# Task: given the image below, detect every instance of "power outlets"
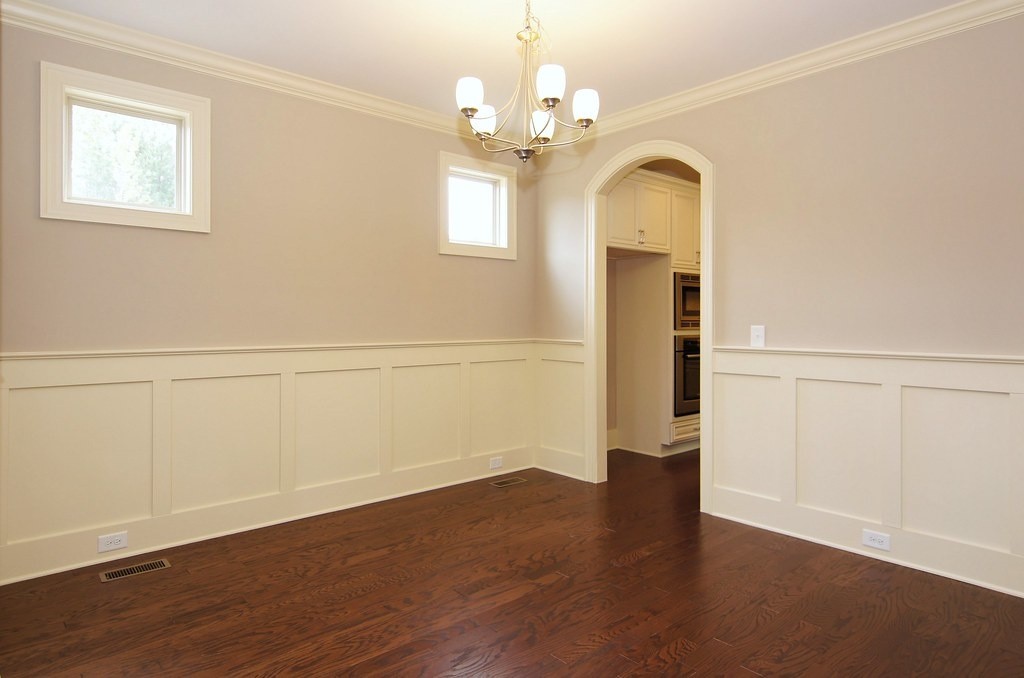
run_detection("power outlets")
[863,529,890,551]
[98,531,128,553]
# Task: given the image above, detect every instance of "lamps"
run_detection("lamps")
[456,0,600,162]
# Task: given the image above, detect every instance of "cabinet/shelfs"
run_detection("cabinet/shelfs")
[607,168,671,259]
[661,414,700,458]
[671,177,700,274]
